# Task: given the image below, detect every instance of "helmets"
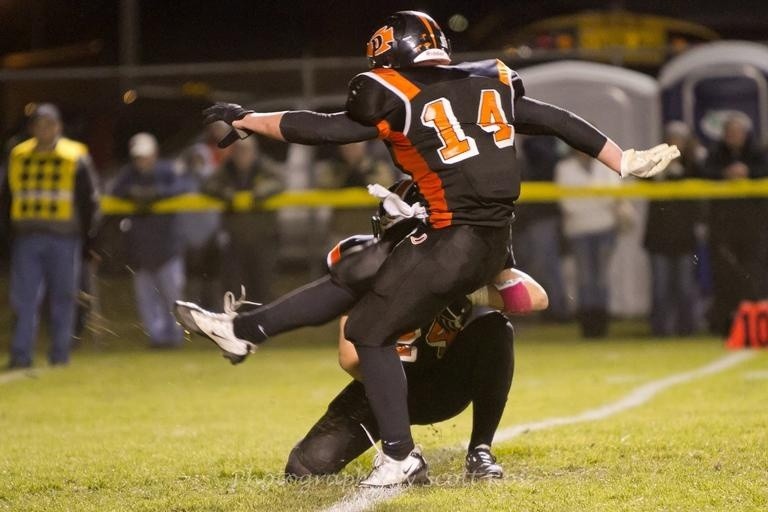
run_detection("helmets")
[364,10,452,67]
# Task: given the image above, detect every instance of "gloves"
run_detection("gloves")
[619,142,681,180]
[200,101,256,150]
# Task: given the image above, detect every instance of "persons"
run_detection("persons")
[555,138,621,340]
[2,101,98,372]
[170,9,681,484]
[703,111,767,337]
[283,175,550,481]
[99,131,199,352]
[220,134,287,304]
[635,119,707,338]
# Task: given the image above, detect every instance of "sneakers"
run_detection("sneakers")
[464,444,505,481]
[170,300,265,367]
[362,442,431,489]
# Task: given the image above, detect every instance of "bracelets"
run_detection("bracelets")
[465,286,490,307]
